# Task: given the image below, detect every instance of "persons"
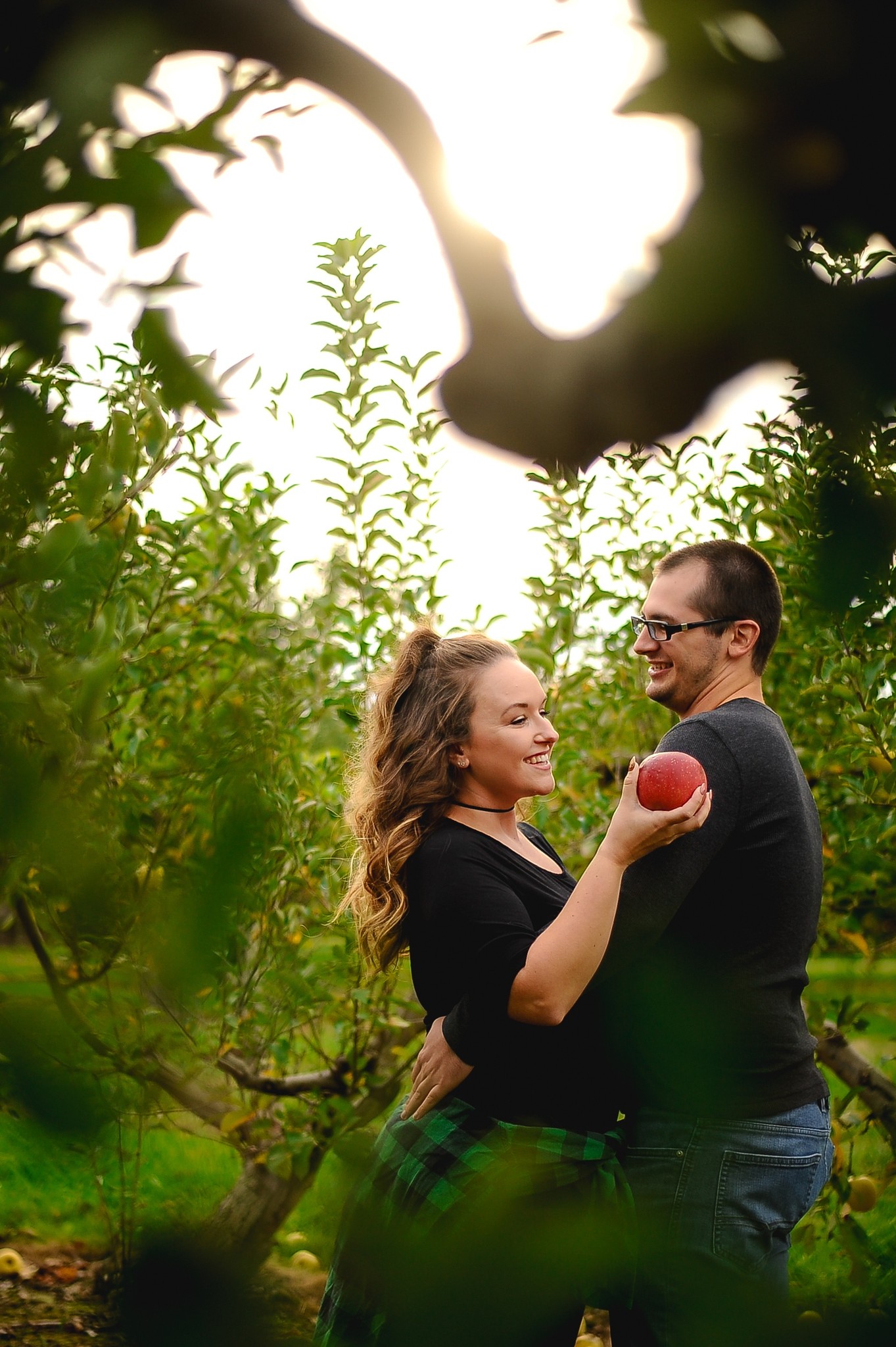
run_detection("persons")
[401,539,836,1347]
[310,629,714,1346]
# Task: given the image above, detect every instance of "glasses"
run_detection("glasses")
[631,616,747,642]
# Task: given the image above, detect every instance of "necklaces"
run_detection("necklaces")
[451,799,517,813]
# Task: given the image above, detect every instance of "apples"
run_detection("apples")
[573,1316,605,1347]
[828,1120,844,1175]
[796,1311,824,1328]
[0,1248,23,1279]
[62,504,138,533]
[846,1176,877,1212]
[135,862,166,891]
[285,1232,318,1273]
[636,752,707,816]
